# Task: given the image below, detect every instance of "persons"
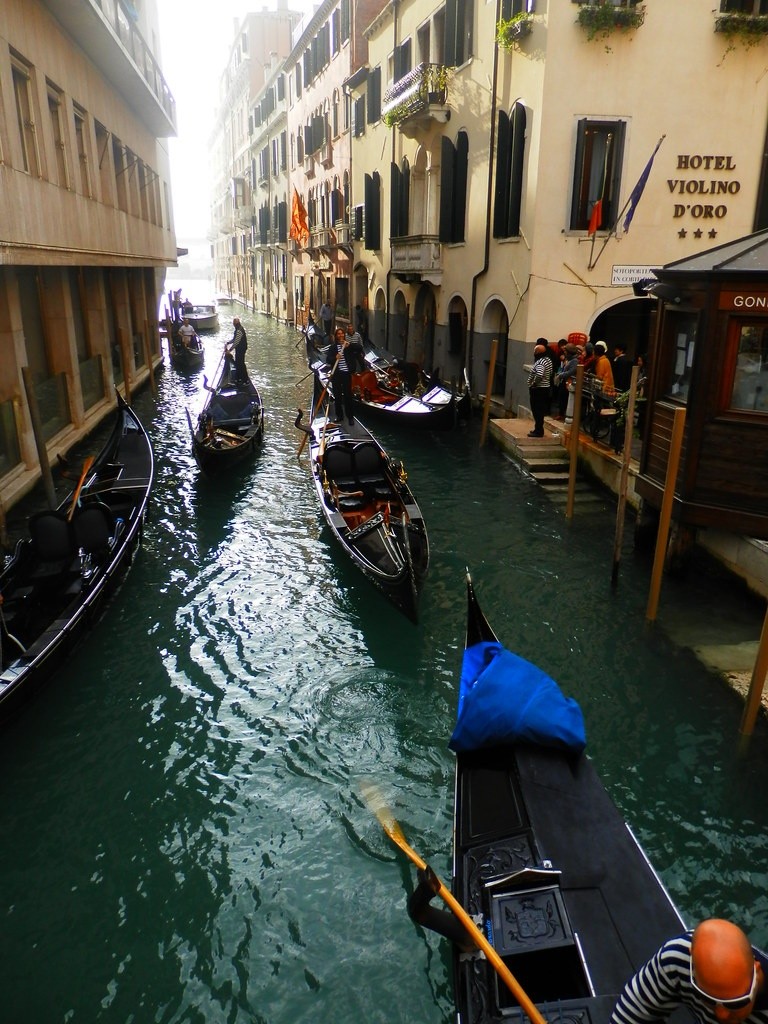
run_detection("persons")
[320,299,333,336]
[356,305,367,337]
[535,338,648,449]
[183,298,193,313]
[226,318,250,386]
[178,319,194,347]
[609,918,768,1024]
[527,345,553,437]
[345,325,363,373]
[326,327,363,426]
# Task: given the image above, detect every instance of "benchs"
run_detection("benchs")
[323,442,392,510]
[28,502,116,579]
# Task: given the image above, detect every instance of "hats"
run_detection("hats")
[576,345,583,354]
[565,343,577,352]
[595,341,608,353]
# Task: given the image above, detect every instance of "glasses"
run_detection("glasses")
[687,953,759,1010]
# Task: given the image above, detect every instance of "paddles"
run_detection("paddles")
[297,341,346,460]
[295,362,327,386]
[390,831,547,1024]
[194,347,227,430]
[295,317,322,348]
[317,404,329,463]
[69,456,95,521]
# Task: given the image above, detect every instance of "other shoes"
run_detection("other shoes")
[553,415,565,420]
[334,417,344,423]
[348,417,355,426]
[241,381,250,387]
[527,430,544,438]
[234,379,242,383]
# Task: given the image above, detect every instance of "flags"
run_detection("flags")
[623,147,657,235]
[588,154,607,236]
[289,189,309,248]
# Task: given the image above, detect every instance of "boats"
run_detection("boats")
[1,386,155,710]
[293,361,433,624]
[191,343,267,466]
[182,301,219,332]
[406,566,700,1024]
[170,330,207,368]
[297,305,476,427]
